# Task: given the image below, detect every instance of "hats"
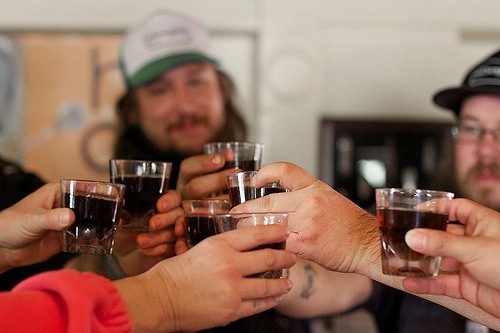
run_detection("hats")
[118,13,237,95]
[431,48,500,117]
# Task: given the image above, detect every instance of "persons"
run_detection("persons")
[0,224,298,333]
[0,153,125,291]
[111,13,500,333]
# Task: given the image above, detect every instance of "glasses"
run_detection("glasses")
[450,124,500,146]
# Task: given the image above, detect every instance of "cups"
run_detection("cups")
[181,198,231,247]
[373,187,454,279]
[58,178,126,256]
[212,210,290,284]
[227,170,288,207]
[108,157,173,233]
[203,142,263,199]
